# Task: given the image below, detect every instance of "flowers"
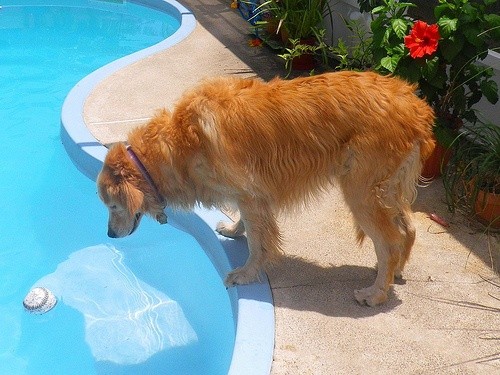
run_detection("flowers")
[230,0,263,48]
[373,0,500,137]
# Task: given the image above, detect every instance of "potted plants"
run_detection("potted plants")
[440,120,500,229]
[265,0,323,71]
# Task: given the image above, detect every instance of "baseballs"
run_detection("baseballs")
[23,286,57,315]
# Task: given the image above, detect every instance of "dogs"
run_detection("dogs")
[97,71,437,307]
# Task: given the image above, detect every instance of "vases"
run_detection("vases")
[419,139,455,180]
